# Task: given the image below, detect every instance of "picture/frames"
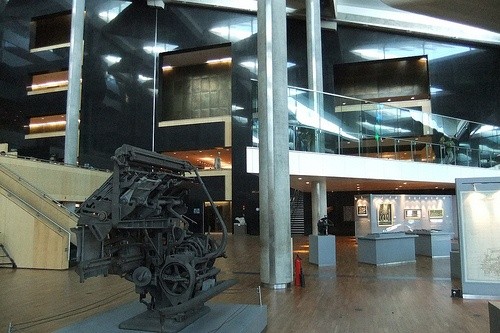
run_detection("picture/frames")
[404,209,423,220]
[459,190,500,284]
[375,202,394,228]
[357,204,368,217]
[428,209,445,219]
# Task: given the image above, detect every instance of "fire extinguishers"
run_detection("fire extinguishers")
[295,256,302,287]
